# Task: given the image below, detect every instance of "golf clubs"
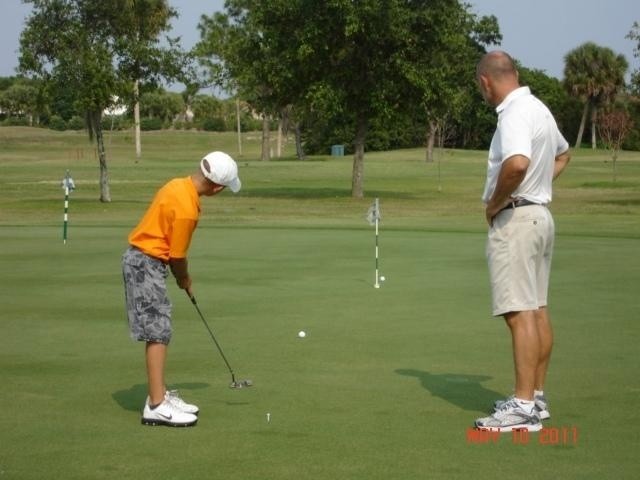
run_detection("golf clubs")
[186,285,253,390]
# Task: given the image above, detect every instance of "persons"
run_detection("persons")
[473,51,572,433]
[121,152,242,427]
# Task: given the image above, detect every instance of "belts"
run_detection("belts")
[503,199,534,209]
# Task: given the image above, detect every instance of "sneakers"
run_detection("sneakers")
[475,400,543,432]
[142,399,198,427]
[145,389,200,414]
[493,394,551,420]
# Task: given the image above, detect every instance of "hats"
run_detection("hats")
[200,151,241,193]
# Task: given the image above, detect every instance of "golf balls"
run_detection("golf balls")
[299,331,306,337]
[380,275,384,281]
